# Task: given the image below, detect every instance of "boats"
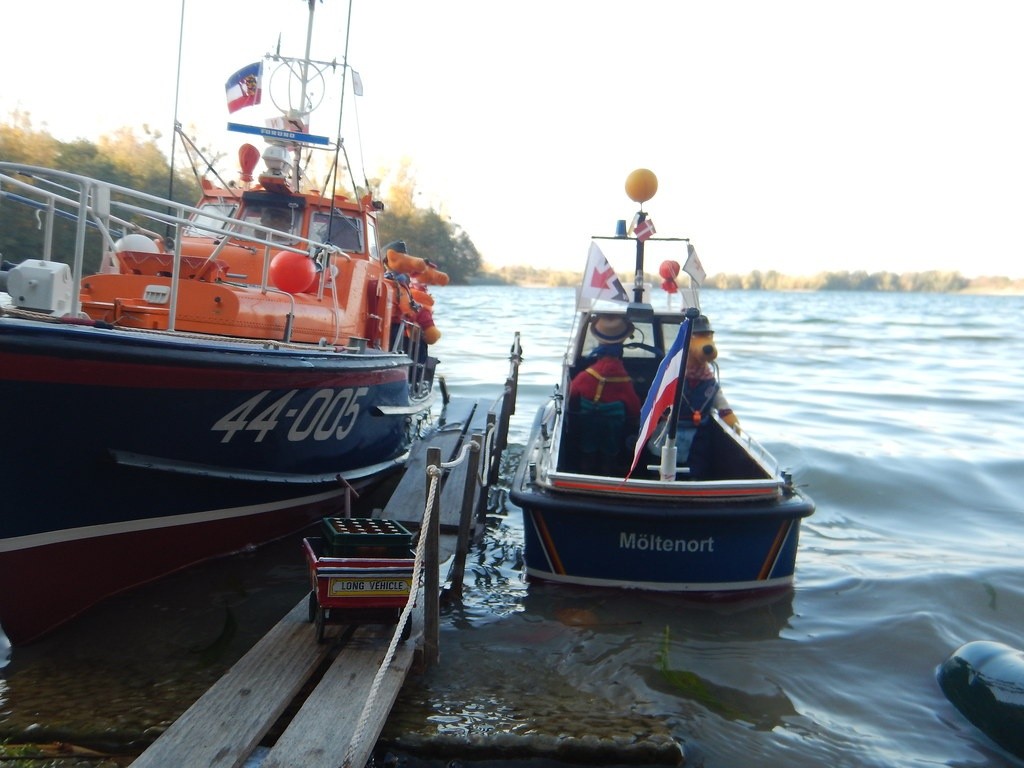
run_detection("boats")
[0,0,444,647]
[508,167,818,601]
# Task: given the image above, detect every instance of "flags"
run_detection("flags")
[684,245,707,286]
[225,62,263,114]
[581,242,633,309]
[622,320,690,485]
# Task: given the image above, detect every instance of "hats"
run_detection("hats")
[380,241,407,262]
[591,314,635,344]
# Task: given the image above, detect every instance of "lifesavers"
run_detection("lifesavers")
[275,115,307,152]
